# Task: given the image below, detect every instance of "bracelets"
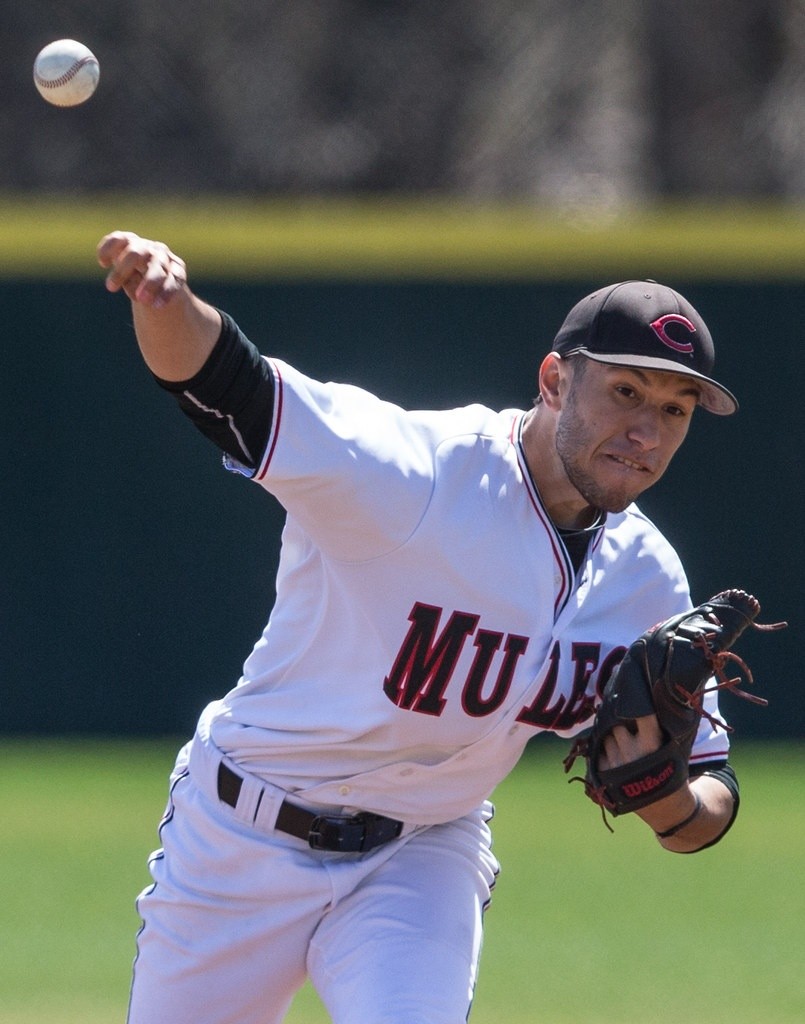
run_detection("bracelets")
[654,784,706,845]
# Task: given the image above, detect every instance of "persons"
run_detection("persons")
[95,227,740,1024]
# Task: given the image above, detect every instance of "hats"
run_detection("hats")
[552,279,739,417]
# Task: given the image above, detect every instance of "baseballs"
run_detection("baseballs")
[33,38,101,107]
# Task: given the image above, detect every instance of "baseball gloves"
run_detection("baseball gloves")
[562,589,788,834]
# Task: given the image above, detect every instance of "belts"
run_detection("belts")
[216,762,404,853]
